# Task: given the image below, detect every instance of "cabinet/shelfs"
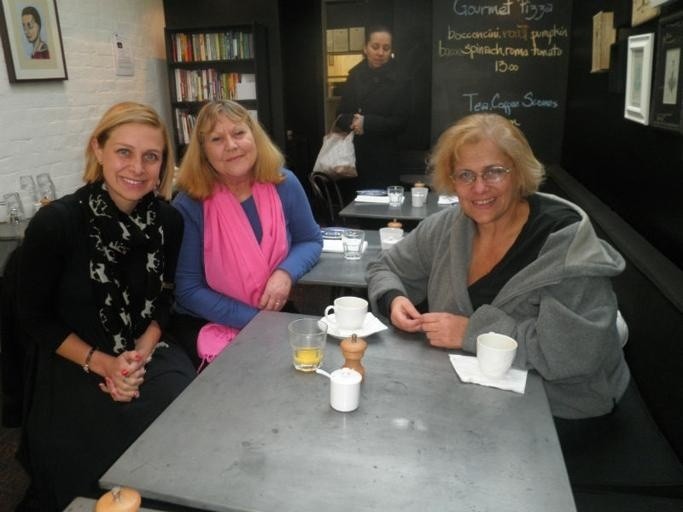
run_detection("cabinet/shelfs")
[162,20,278,171]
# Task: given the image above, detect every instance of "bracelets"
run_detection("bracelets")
[82,345,96,375]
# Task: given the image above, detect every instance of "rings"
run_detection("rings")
[273,301,282,307]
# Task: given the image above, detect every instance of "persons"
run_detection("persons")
[364,113,631,484]
[166,100,323,367]
[3,101,197,497]
[19,5,51,60]
[335,20,416,188]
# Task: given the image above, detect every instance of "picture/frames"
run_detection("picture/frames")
[623,31,654,127]
[652,11,682,131]
[1,0,71,87]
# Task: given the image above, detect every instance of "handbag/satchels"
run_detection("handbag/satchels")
[311,112,360,180]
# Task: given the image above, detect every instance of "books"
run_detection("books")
[173,29,257,146]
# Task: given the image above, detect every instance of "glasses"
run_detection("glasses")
[447,161,517,184]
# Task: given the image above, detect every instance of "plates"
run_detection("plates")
[318,315,374,340]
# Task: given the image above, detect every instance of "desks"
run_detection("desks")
[336,191,459,234]
[295,225,414,310]
[96,308,579,512]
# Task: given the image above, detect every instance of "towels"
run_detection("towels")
[316,234,376,260]
[354,193,404,206]
[434,192,460,207]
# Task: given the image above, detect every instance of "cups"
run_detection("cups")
[330,367,363,412]
[288,317,329,371]
[475,332,519,379]
[323,295,368,331]
[340,230,365,260]
[387,185,403,206]
[413,188,430,207]
[379,227,404,252]
